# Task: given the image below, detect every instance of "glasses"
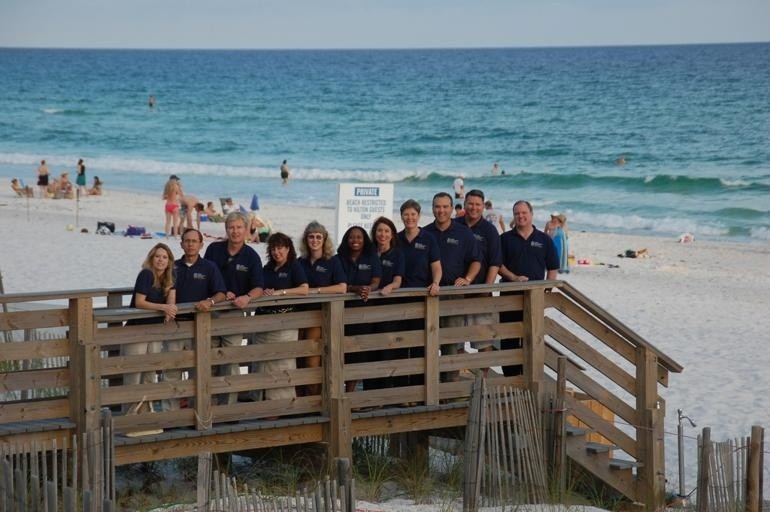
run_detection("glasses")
[308,235,324,240]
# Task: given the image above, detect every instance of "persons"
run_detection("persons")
[11,158,103,199]
[618,247,648,258]
[149,93,154,111]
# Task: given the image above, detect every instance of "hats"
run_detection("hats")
[169,174,180,180]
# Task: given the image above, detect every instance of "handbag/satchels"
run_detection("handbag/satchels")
[121,395,164,438]
